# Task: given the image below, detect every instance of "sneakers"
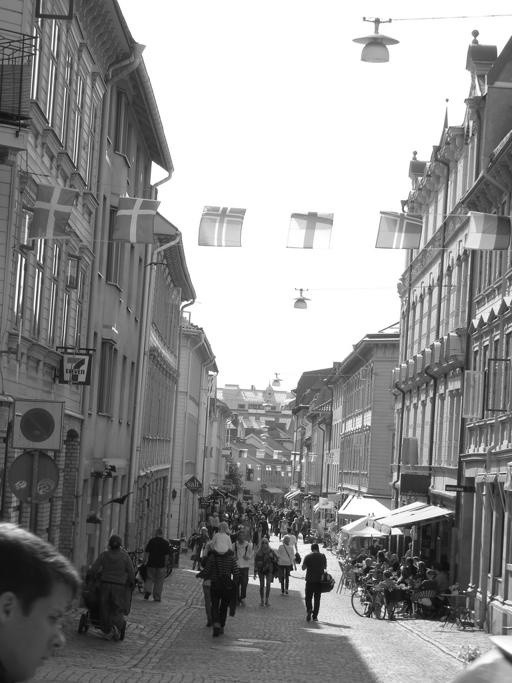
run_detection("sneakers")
[207,622,212,627]
[214,626,220,637]
[306,609,318,621]
[144,592,151,599]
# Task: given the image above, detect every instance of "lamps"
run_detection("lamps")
[294,284,456,309]
[352,13,511,63]
[272,372,355,387]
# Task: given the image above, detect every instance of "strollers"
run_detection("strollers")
[76,565,128,644]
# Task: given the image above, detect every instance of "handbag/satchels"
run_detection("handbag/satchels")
[320,573,335,592]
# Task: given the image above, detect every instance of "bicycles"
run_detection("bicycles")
[164,543,181,579]
[349,575,389,620]
[329,539,346,560]
[127,547,151,593]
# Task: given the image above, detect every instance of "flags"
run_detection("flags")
[112,193,160,244]
[466,212,509,249]
[375,209,428,252]
[29,184,77,241]
[286,207,337,253]
[196,203,249,247]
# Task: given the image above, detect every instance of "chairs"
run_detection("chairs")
[336,553,474,631]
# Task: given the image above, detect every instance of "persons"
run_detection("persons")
[188,499,451,634]
[88,535,136,640]
[140,528,171,603]
[0,523,83,683]
